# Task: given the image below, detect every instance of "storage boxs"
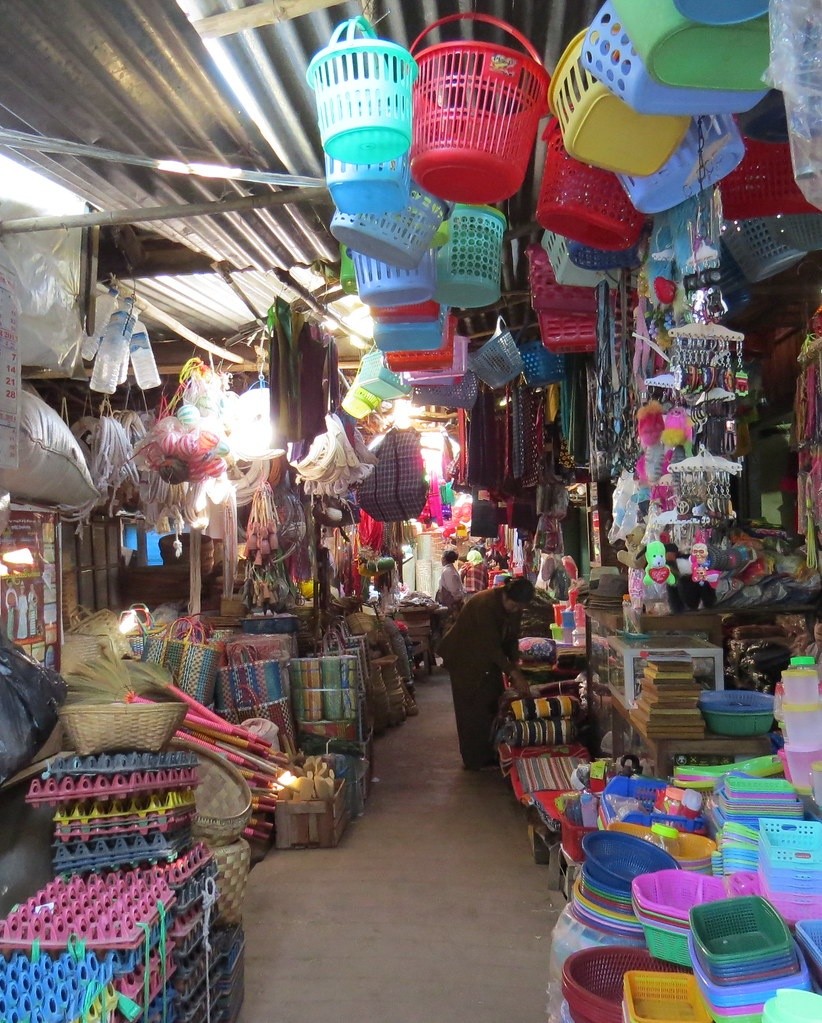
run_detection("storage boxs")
[266,778,351,848]
[607,633,724,712]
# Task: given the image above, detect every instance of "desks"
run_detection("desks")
[583,607,723,721]
[609,695,771,779]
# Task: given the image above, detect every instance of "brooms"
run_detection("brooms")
[62,647,293,842]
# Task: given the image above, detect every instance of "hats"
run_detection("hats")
[580,563,628,611]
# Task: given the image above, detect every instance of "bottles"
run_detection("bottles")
[642,823,680,857]
[580,795,598,827]
[81,289,161,394]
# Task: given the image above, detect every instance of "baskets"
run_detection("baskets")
[552,691,822,1023]
[62,632,104,673]
[58,699,188,757]
[163,735,251,850]
[303,0,822,420]
[209,839,251,927]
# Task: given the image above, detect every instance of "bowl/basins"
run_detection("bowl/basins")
[778,669,822,805]
[701,710,774,736]
[241,617,294,633]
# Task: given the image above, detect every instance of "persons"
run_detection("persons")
[464,549,489,603]
[437,574,536,774]
[469,539,492,569]
[438,550,465,668]
[317,541,338,582]
[496,549,511,570]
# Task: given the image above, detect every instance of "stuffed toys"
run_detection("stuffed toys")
[643,540,677,586]
[633,401,696,511]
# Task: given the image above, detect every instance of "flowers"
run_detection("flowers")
[519,638,552,658]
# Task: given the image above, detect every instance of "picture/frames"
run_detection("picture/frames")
[0,572,45,645]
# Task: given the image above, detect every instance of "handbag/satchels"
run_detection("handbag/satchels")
[354,425,430,525]
[312,497,359,529]
[440,587,465,607]
[69,603,373,743]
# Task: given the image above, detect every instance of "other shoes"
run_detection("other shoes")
[461,755,501,771]
